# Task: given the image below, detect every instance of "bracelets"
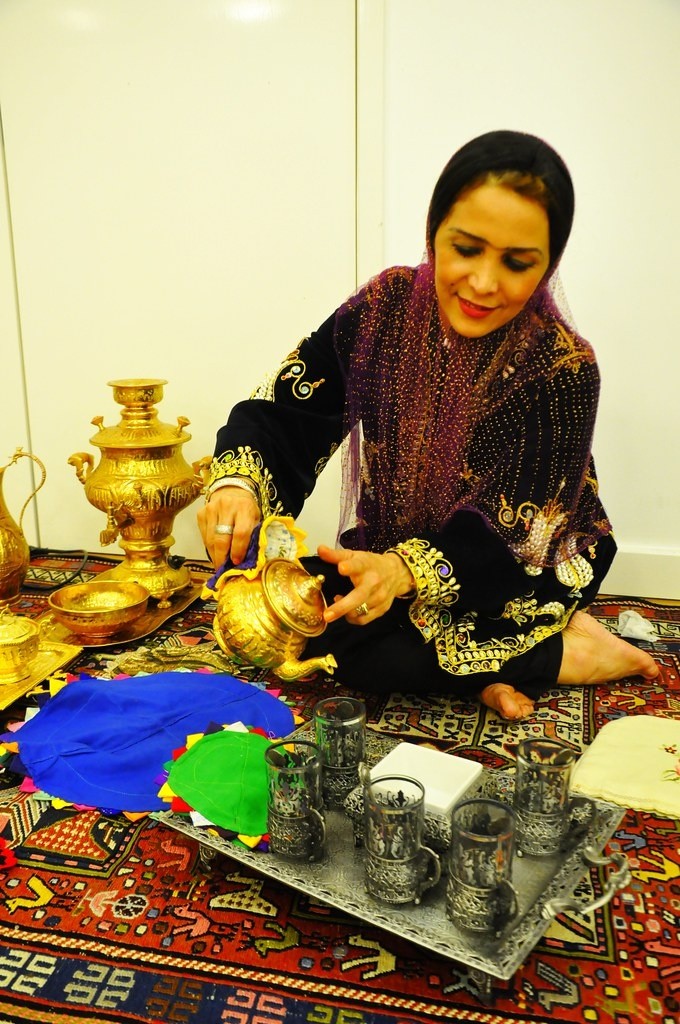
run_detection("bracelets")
[205,477,259,504]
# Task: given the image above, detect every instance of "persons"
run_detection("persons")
[196,132,660,722]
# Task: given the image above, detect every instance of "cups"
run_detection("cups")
[446,800,518,933]
[314,697,367,812]
[515,739,596,855]
[364,775,440,906]
[265,740,327,862]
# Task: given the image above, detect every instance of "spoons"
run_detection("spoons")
[269,749,288,802]
[554,748,574,777]
[334,701,354,721]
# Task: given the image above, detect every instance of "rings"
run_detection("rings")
[214,525,233,536]
[355,603,368,617]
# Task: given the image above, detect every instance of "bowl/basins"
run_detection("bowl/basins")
[49,585,149,637]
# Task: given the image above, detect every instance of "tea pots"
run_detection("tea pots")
[213,559,337,681]
